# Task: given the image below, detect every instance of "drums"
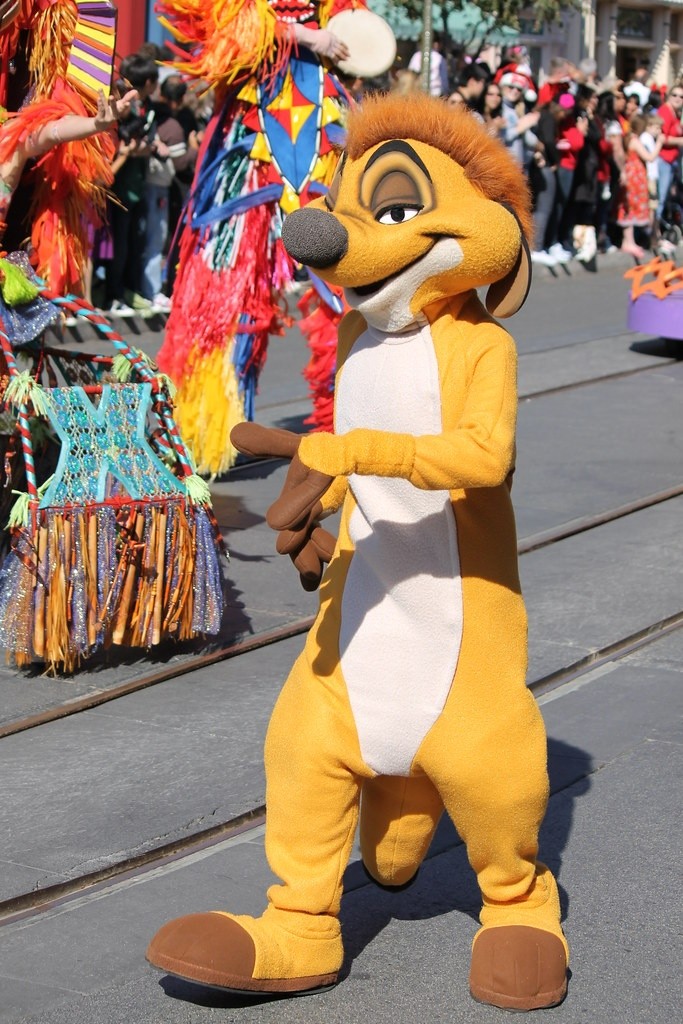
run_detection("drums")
[326,8,398,79]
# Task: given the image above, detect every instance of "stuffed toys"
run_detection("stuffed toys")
[147,97,571,1010]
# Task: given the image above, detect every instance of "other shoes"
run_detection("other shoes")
[531,242,572,267]
[621,244,645,257]
[110,290,172,318]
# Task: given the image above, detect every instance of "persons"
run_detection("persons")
[78,30,683,316]
[154,0,366,375]
[0,0,141,302]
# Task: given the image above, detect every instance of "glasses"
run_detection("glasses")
[508,85,522,91]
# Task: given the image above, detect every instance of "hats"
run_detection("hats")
[498,64,537,102]
